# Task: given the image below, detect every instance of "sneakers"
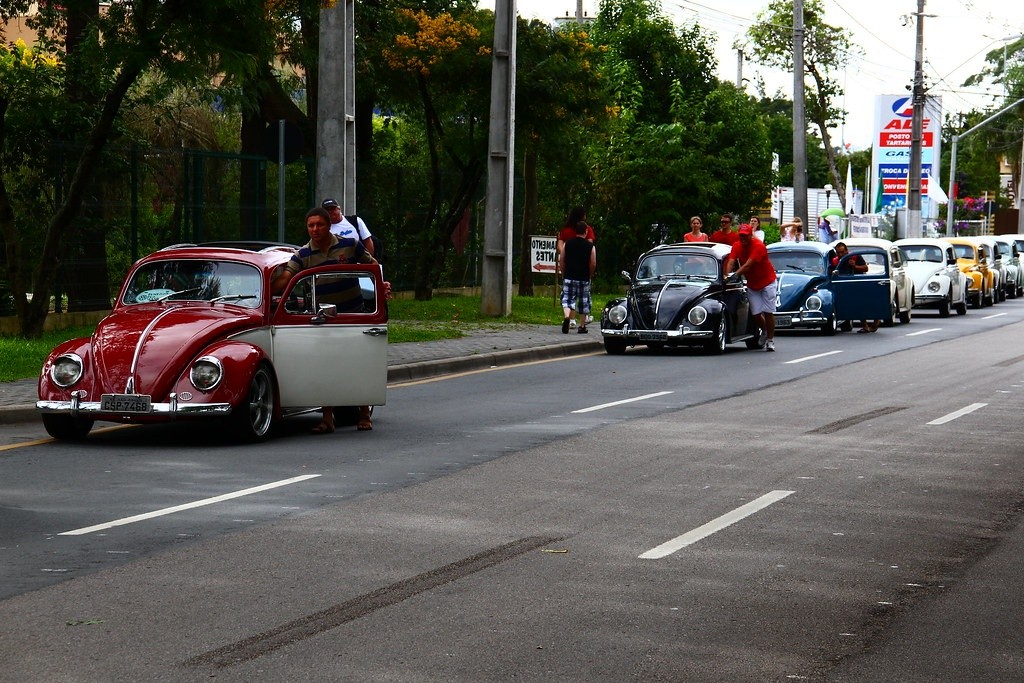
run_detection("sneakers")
[758,331,768,345]
[767,341,775,351]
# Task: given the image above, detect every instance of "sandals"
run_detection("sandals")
[357,415,372,430]
[310,421,335,434]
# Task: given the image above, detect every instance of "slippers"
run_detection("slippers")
[857,328,870,333]
[870,321,881,332]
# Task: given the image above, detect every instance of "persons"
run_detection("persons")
[684,217,708,263]
[819,218,838,244]
[558,206,594,329]
[560,221,596,334]
[710,215,738,248]
[829,243,880,332]
[747,217,766,246]
[779,217,804,243]
[268,199,391,432]
[724,224,777,351]
[320,199,374,262]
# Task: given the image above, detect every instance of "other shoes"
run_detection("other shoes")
[562,317,570,333]
[569,322,575,328]
[586,316,593,325]
[578,326,588,333]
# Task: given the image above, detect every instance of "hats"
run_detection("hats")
[738,224,752,235]
[322,199,339,208]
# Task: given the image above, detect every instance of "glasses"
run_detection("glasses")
[721,220,731,224]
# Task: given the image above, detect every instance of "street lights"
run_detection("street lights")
[824,185,833,209]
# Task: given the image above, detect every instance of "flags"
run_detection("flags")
[845,164,853,214]
[927,174,948,204]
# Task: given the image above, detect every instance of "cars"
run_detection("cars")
[600,241,765,356]
[35,239,387,443]
[763,240,891,336]
[829,234,1024,324]
